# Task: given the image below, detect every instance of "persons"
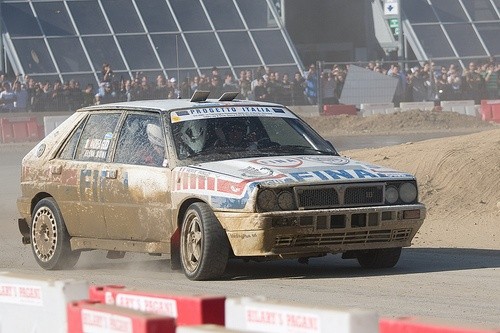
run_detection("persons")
[127,128,169,168]
[214,123,268,157]
[2,57,500,108]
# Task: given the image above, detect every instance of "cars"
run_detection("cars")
[16,89,427,281]
